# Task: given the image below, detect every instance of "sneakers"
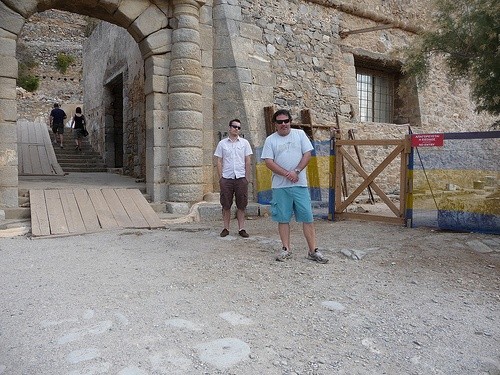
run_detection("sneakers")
[307,248,329,264]
[276,246,293,261]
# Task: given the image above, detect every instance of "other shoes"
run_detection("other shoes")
[220,229,229,237]
[79,148,82,151]
[239,229,249,237]
[53,140,58,144]
[73,145,79,151]
[60,144,64,148]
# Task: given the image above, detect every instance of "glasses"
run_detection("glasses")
[275,118,290,124]
[229,125,241,130]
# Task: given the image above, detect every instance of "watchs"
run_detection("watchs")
[294,168,300,173]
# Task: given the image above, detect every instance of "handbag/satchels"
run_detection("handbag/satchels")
[80,123,89,137]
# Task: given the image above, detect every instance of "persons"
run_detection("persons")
[49,103,67,148]
[261,109,329,264]
[214,119,253,238]
[70,106,86,151]
